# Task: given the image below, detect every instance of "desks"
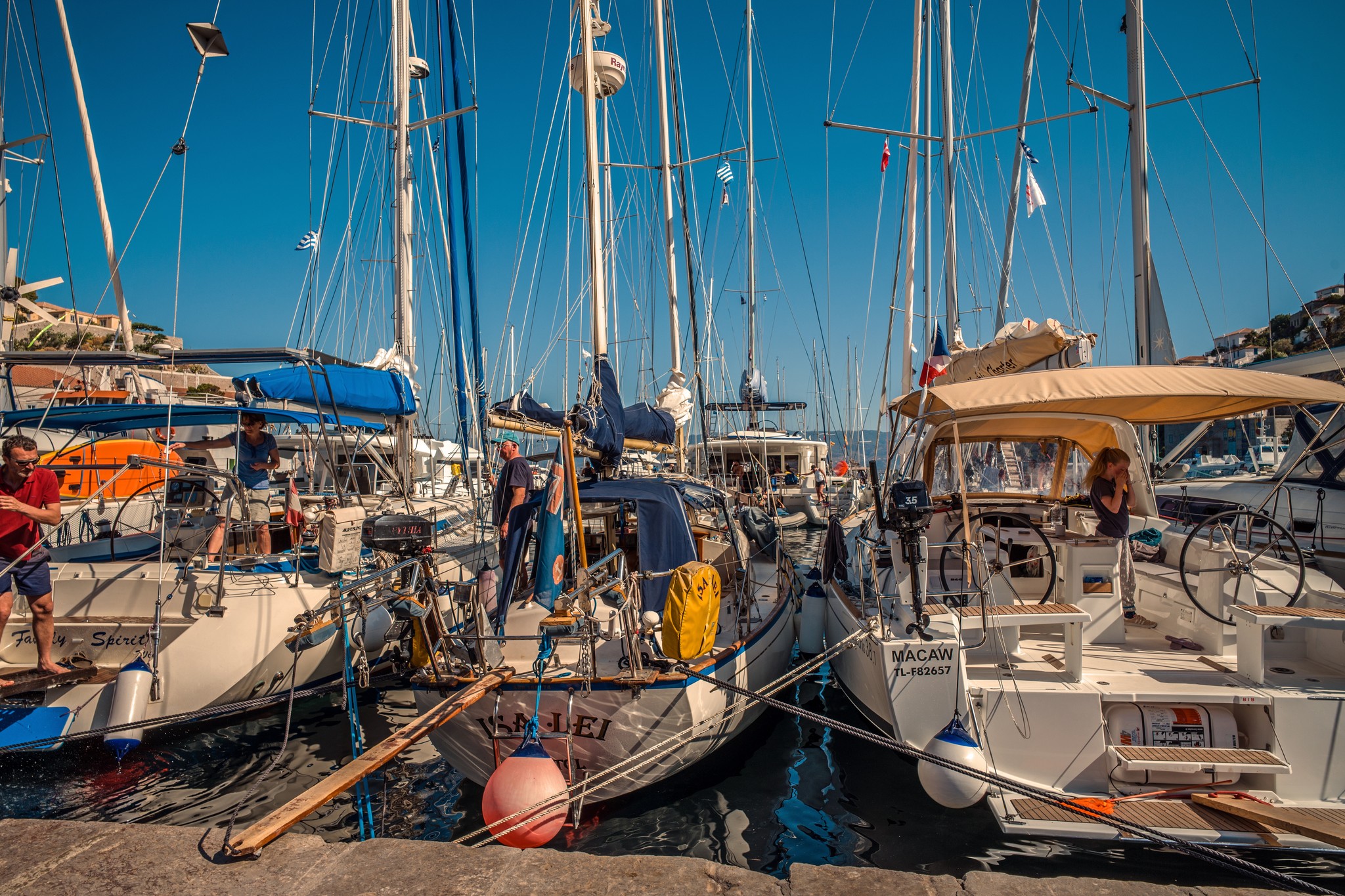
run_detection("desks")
[1039,526,1127,647]
[977,525,1066,604]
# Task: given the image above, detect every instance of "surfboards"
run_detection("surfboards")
[36,438,185,499]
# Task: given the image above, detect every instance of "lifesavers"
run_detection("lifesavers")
[156,426,174,440]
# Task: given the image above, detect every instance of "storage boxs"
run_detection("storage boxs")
[357,513,434,556]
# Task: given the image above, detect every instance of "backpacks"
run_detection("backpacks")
[1060,492,1093,509]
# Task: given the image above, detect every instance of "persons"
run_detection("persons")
[734,501,745,519]
[169,412,281,564]
[785,465,794,474]
[490,433,535,595]
[581,461,595,478]
[799,465,827,506]
[754,486,785,516]
[0,434,71,686]
[857,470,867,484]
[653,466,659,472]
[1080,446,1158,628]
[732,461,746,494]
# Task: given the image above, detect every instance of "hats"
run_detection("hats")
[490,431,520,447]
[770,496,774,503]
[739,501,745,504]
[735,460,741,464]
[811,465,815,470]
[775,468,781,472]
[785,465,791,468]
[754,487,763,494]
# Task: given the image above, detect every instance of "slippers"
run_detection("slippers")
[1164,634,1202,651]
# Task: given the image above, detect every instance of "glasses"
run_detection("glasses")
[8,453,41,467]
[240,420,262,427]
[495,444,511,449]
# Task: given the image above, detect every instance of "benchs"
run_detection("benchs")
[1232,600,1345,691]
[950,603,1092,689]
[890,585,961,642]
[1129,555,1201,644]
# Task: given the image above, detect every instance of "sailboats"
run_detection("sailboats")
[2,2,1345,884]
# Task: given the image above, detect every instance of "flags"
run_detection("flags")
[295,231,317,250]
[717,161,734,186]
[880,140,890,172]
[919,318,952,388]
[1018,137,1039,164]
[286,477,304,526]
[533,434,565,613]
[1026,166,1046,219]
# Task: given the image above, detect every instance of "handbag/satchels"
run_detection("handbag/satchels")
[1129,540,1162,563]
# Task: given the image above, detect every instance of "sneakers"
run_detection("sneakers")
[816,502,822,505]
[1123,613,1158,632]
[823,500,826,506]
